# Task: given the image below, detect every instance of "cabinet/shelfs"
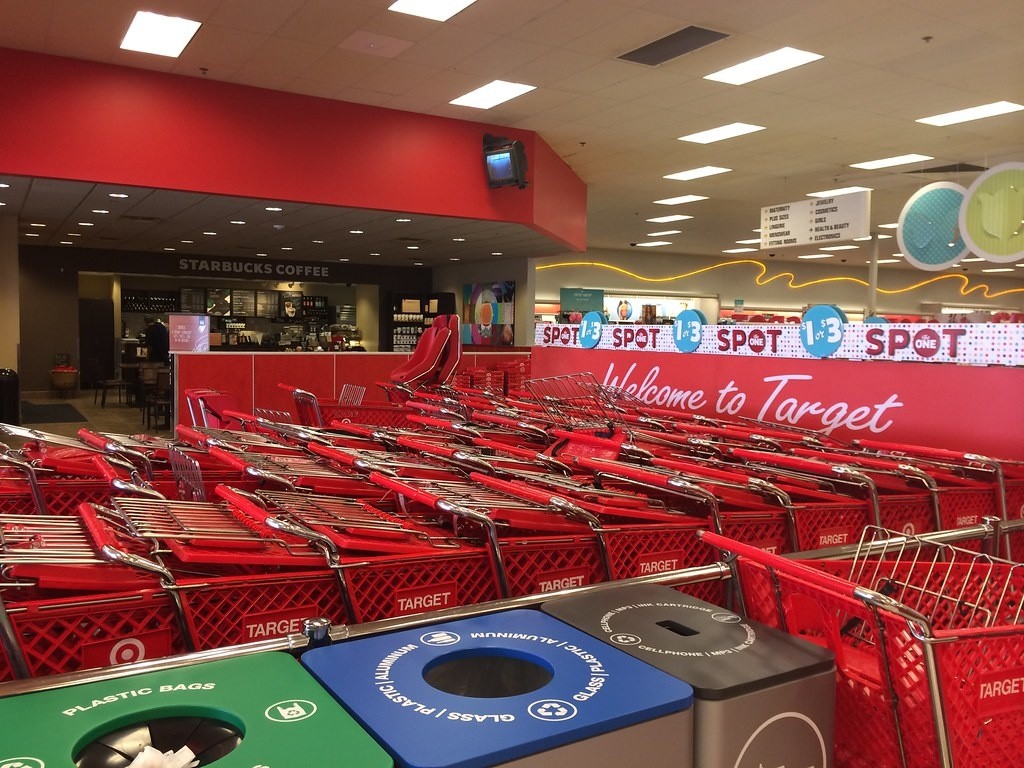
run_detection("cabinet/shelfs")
[391,292,456,351]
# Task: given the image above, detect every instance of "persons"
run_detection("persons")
[619,300,629,320]
[144,314,171,366]
[603,310,609,321]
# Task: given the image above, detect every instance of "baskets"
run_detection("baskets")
[445,358,531,396]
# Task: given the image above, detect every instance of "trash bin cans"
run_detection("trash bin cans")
[299,607,696,768]
[541,581,837,768]
[0,650,396,768]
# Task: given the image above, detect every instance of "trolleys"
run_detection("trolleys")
[694,528,1024,768]
[1,370,1024,680]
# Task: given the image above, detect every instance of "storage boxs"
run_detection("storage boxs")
[402,298,420,312]
[429,299,437,313]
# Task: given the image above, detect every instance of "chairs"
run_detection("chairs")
[88,357,125,407]
[145,372,171,433]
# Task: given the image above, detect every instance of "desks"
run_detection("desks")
[116,362,171,411]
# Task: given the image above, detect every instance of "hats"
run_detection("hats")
[144,314,153,322]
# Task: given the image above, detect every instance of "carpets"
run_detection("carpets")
[23,403,88,425]
